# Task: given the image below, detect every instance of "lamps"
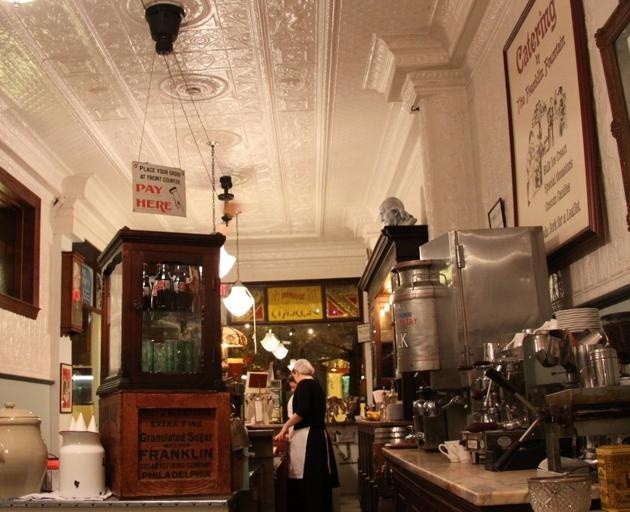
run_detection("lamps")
[208,141,237,279]
[259,328,289,361]
[224,207,254,316]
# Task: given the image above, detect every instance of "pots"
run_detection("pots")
[0,399,49,501]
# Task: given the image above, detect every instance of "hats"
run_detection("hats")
[294,359,315,374]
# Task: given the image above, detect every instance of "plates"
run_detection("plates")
[553,308,602,334]
[388,403,404,420]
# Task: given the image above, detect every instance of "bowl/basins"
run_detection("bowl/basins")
[527,474,592,511]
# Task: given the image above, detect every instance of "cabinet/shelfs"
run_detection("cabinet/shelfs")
[96,225,226,399]
[328,426,360,494]
[383,458,531,512]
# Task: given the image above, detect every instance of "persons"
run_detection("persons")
[62,379,70,409]
[270,358,334,511]
[378,198,418,226]
[286,374,342,489]
[168,186,185,214]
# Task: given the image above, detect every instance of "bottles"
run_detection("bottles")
[142,263,192,313]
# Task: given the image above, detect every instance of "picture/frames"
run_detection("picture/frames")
[594,0,630,232]
[59,363,74,413]
[487,197,506,229]
[502,0,602,264]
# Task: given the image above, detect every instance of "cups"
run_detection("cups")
[584,348,619,388]
[438,440,475,464]
[59,431,106,498]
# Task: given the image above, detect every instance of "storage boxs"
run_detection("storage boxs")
[97,393,233,498]
